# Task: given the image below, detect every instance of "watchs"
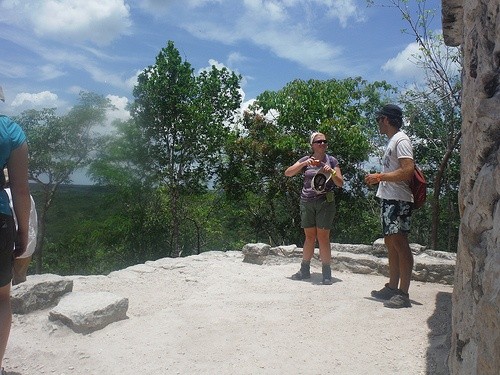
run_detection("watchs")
[375,172,382,182]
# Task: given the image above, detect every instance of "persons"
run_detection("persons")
[0,116,38,375]
[285,133,344,285]
[365,104,414,308]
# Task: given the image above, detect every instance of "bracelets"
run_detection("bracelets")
[331,170,337,177]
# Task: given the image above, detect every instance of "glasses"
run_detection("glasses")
[376,116,385,122]
[312,140,327,144]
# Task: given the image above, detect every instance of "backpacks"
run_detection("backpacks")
[410,165,426,205]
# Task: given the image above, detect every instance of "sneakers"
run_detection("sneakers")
[371,284,399,299]
[291,268,311,280]
[323,265,331,285]
[383,294,411,308]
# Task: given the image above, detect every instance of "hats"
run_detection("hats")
[375,104,402,117]
[311,167,332,193]
